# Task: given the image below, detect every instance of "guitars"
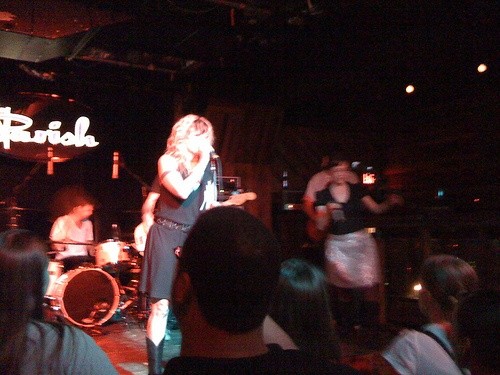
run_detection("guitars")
[134,192,257,256]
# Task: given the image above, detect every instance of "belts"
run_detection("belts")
[154,216,193,233]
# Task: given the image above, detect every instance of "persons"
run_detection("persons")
[139,169,167,230]
[312,150,406,352]
[0,195,500,375]
[300,146,361,220]
[138,111,218,375]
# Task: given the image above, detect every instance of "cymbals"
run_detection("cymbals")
[47,239,93,245]
[46,250,67,255]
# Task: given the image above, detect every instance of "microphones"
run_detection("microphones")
[47,142,53,175]
[210,151,218,160]
[112,151,119,179]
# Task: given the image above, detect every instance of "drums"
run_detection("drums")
[95,239,131,273]
[51,266,120,328]
[43,259,64,300]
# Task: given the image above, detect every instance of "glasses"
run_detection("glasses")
[173,246,195,277]
